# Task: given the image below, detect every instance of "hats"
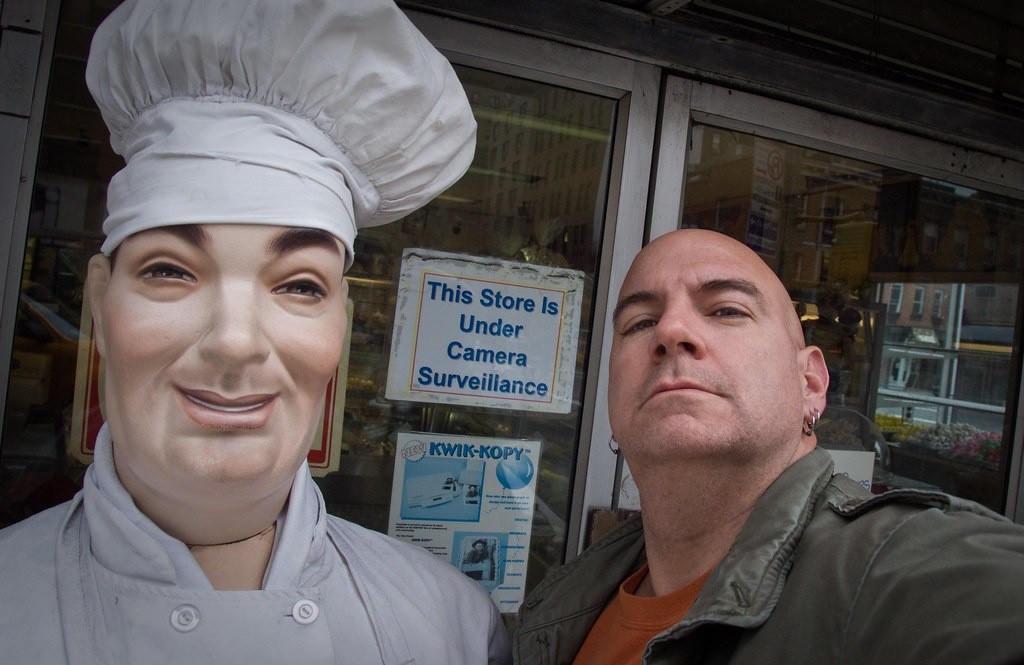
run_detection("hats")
[84,0,477,274]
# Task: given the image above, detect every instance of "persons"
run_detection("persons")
[796,290,861,391]
[1,0,507,665]
[507,228,1022,665]
